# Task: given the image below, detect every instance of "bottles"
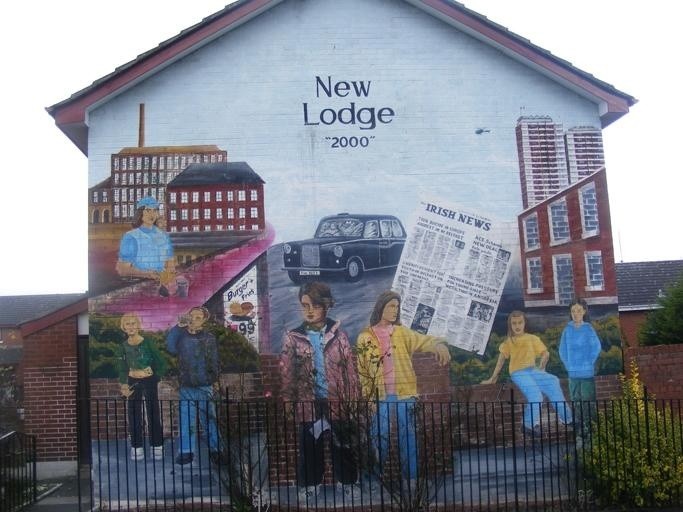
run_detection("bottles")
[121,382,137,400]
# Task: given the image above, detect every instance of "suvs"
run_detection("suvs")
[282,212,407,285]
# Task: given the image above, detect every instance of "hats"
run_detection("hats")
[137,198,161,209]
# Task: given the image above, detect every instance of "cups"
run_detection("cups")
[178,314,192,324]
[177,279,188,297]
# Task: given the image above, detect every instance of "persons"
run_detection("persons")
[164,305,224,463]
[113,193,176,282]
[279,282,360,503]
[559,298,601,424]
[481,311,570,434]
[356,291,450,490]
[114,315,164,458]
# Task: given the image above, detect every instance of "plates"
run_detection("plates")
[229,311,256,322]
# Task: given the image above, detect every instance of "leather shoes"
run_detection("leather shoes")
[521,424,542,437]
